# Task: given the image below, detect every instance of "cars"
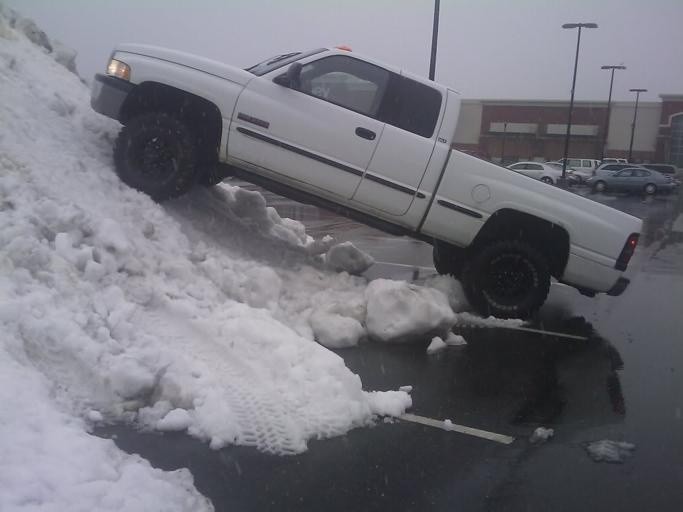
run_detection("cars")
[502,155,682,195]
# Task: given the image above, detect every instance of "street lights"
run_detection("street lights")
[558,21,646,195]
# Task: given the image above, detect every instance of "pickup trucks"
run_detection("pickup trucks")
[91,33,647,320]
